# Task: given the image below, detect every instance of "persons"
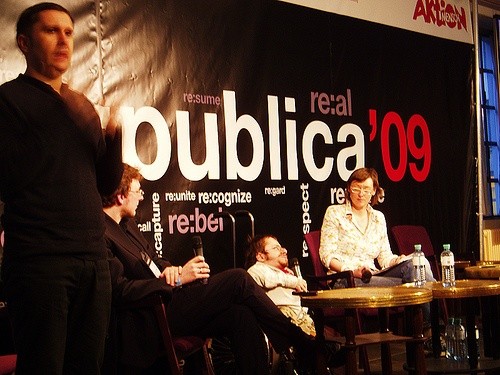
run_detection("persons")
[0,2,112,375]
[318,168,449,357]
[245,235,316,337]
[100,162,343,375]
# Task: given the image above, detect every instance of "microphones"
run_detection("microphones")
[361,268,372,284]
[191,236,208,284]
[290,258,302,279]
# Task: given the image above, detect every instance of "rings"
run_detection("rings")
[199,268,203,274]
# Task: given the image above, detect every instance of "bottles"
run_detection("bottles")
[445,317,479,361]
[441,244,456,288]
[412,245,426,287]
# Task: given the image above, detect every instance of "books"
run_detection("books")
[371,252,424,276]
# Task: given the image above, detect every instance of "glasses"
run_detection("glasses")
[350,187,373,194]
[128,190,144,196]
[264,246,280,252]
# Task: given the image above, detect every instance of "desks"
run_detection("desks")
[401,280,500,375]
[301,287,433,375]
[465,264,500,278]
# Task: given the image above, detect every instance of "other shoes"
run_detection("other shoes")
[425,334,450,357]
[299,347,354,369]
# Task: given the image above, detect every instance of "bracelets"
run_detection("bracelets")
[175,278,182,287]
[341,265,349,272]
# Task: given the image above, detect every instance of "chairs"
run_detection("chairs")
[305,212,449,375]
[110,295,217,375]
